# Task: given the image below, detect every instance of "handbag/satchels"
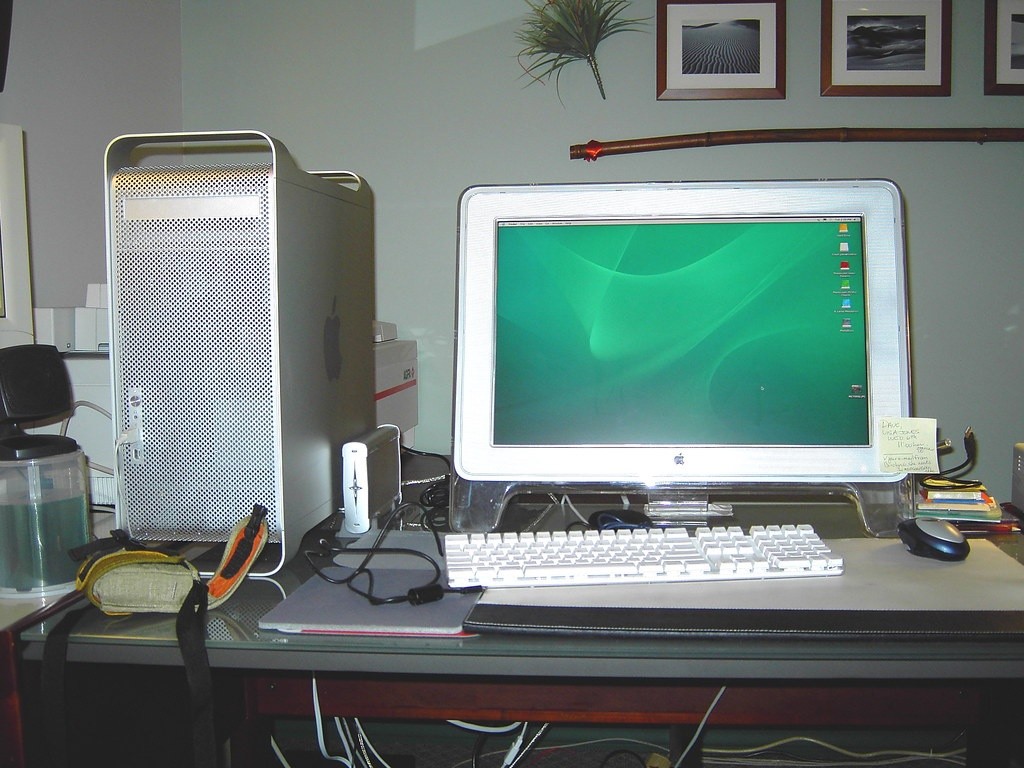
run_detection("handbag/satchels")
[68,528,201,615]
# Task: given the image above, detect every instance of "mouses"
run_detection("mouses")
[895,515,971,561]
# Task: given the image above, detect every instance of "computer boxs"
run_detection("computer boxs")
[104,132,376,576]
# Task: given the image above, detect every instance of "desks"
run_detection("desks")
[0,455,1024,768]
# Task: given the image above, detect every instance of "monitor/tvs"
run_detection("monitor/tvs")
[444,181,914,535]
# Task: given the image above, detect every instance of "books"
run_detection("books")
[914,489,1002,522]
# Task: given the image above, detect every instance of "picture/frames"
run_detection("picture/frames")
[820,0,952,97]
[656,0,786,100]
[984,0,1024,95]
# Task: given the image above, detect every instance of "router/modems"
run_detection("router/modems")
[342,422,403,533]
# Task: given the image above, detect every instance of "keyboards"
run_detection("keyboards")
[444,524,842,588]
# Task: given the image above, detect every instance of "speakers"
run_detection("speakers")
[0,343,78,462]
